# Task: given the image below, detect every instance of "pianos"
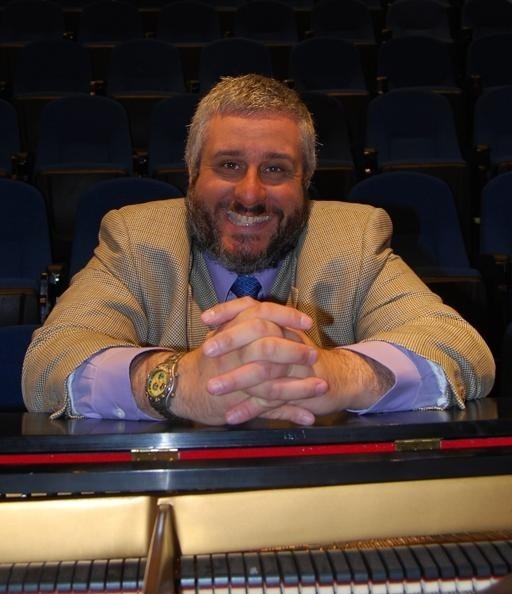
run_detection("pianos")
[1,395,511,594]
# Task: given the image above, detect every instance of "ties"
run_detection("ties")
[230,277,262,300]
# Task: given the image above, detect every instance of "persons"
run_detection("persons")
[21,72,497,427]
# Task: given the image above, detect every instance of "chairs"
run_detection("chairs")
[0,1,511,414]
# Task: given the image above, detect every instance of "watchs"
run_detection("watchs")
[146,350,191,424]
